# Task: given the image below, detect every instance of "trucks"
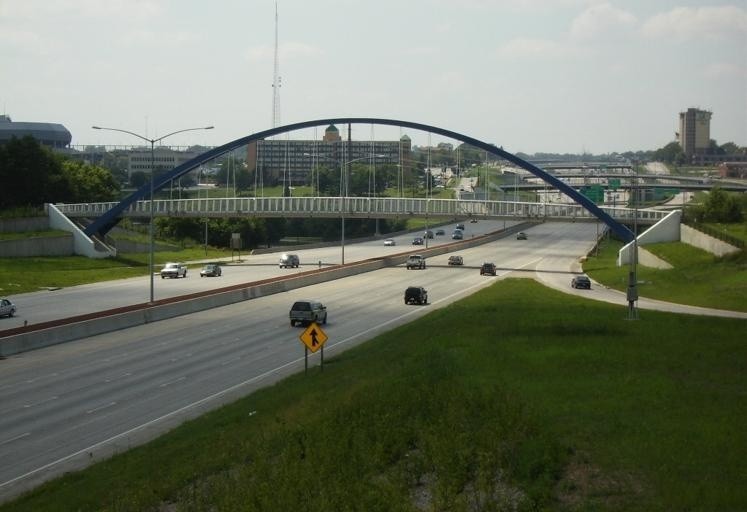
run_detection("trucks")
[160,262,188,279]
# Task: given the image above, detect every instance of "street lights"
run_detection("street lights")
[92,125,215,302]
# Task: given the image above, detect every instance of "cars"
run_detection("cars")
[384,224,528,246]
[404,287,427,304]
[200,264,222,277]
[480,263,497,276]
[279,254,299,268]
[289,298,327,327]
[448,255,463,265]
[406,255,426,269]
[571,276,590,289]
[0,299,16,318]
